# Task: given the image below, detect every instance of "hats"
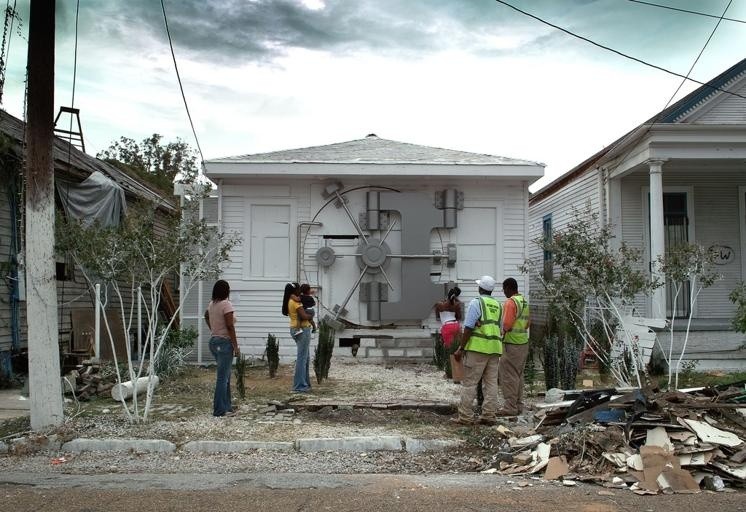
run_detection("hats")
[475,276,495,292]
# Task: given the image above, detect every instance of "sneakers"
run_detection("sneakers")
[449,417,473,426]
[312,329,317,333]
[496,409,522,416]
[294,328,304,336]
[473,419,496,426]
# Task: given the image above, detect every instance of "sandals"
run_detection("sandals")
[222,405,241,417]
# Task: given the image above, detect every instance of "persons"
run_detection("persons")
[495,278,530,416]
[436,287,462,379]
[205,280,240,416]
[450,276,505,425]
[282,282,317,392]
